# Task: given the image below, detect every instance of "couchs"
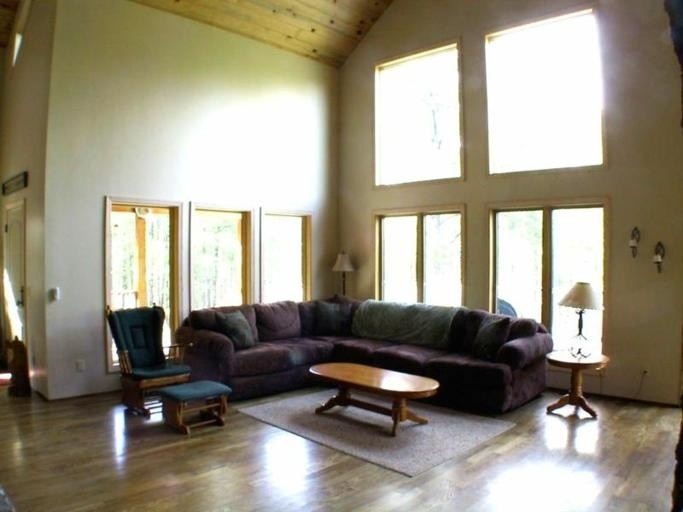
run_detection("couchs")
[176,299,553,415]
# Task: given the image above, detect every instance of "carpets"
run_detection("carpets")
[238,388,517,477]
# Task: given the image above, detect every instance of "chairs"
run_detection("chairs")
[104,305,193,416]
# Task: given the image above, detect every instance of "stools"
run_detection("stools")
[157,380,233,434]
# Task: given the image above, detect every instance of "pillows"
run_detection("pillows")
[315,301,353,335]
[509,318,537,338]
[475,315,510,362]
[216,311,255,350]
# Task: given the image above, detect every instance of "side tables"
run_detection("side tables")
[546,352,609,418]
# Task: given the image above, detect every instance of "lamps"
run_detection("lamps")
[332,251,355,295]
[628,226,639,258]
[559,281,605,358]
[652,242,665,274]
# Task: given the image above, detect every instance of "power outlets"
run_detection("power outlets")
[640,368,649,376]
